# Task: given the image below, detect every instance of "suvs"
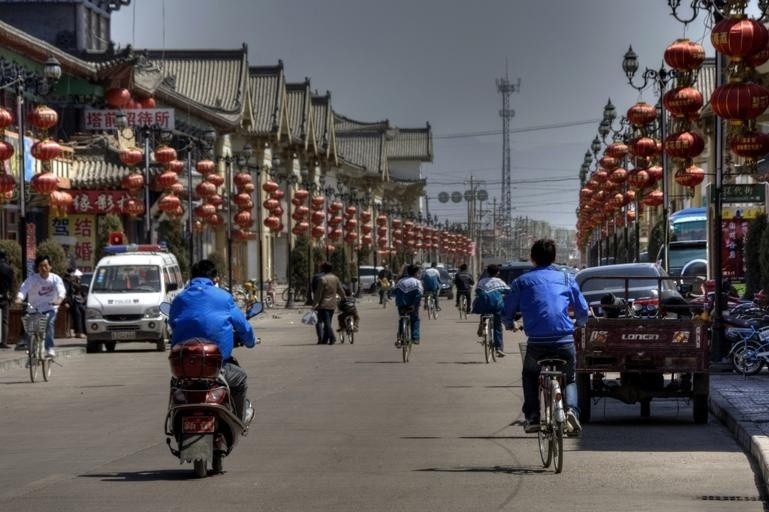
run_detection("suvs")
[657,240,711,298]
[358,264,396,293]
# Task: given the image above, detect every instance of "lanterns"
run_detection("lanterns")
[1,97,286,235]
[662,86,704,121]
[665,131,705,165]
[580,102,663,243]
[663,38,706,72]
[292,190,472,257]
[725,127,765,166]
[709,77,764,136]
[711,15,763,65]
[674,160,705,197]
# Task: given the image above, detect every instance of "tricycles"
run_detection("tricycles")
[576,276,711,422]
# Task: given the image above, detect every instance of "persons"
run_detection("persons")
[63,268,87,338]
[395,264,424,348]
[312,261,347,344]
[471,264,512,357]
[168,260,255,426]
[312,262,346,345]
[336,288,359,332]
[377,264,393,304]
[0,248,16,348]
[422,262,443,311]
[15,254,67,357]
[455,264,474,314]
[501,239,583,433]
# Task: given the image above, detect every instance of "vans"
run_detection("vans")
[84,242,185,353]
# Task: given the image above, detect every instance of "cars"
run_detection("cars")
[401,262,461,300]
[573,263,692,320]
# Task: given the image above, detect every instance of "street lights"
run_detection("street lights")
[278,174,473,308]
[0,52,61,281]
[115,112,172,246]
[243,142,275,307]
[578,97,661,266]
[668,1,768,367]
[161,126,216,267]
[622,45,694,274]
[206,148,247,292]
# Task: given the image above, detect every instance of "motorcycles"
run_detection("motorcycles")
[722,290,769,375]
[165,337,255,477]
[231,278,295,308]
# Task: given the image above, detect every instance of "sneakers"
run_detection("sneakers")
[44,349,56,358]
[565,408,583,433]
[524,419,541,433]
[244,406,255,426]
[0,342,12,349]
[25,357,34,369]
[495,350,506,358]
[76,333,87,339]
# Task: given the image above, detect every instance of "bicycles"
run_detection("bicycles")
[336,296,360,344]
[395,306,420,362]
[15,300,57,382]
[378,278,392,308]
[515,325,578,473]
[478,313,503,363]
